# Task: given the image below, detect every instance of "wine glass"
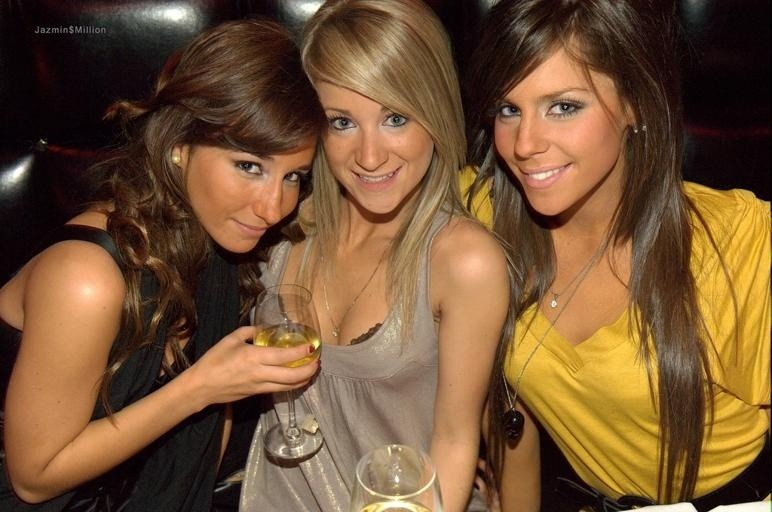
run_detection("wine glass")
[347,441,437,511]
[253,284,324,460]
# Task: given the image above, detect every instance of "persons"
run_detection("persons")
[0,0,772,511]
